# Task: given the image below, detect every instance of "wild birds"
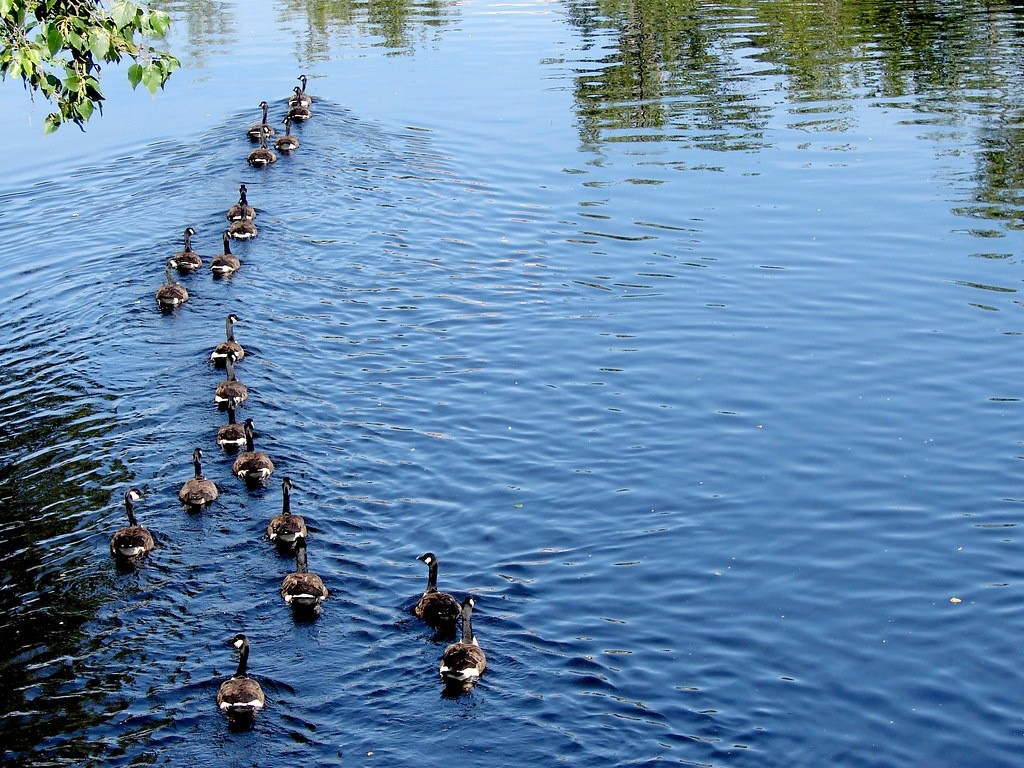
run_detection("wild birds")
[281,535,329,607]
[157,257,188,304]
[216,634,266,712]
[211,231,241,274]
[227,184,256,221]
[276,117,299,150]
[226,198,258,238]
[288,74,313,119]
[216,397,248,449]
[210,314,245,364]
[214,349,247,410]
[248,101,275,140]
[178,447,219,507]
[232,418,273,480]
[172,226,203,270]
[268,476,306,550]
[110,489,153,557]
[247,125,276,167]
[439,599,486,686]
[414,550,463,624]
